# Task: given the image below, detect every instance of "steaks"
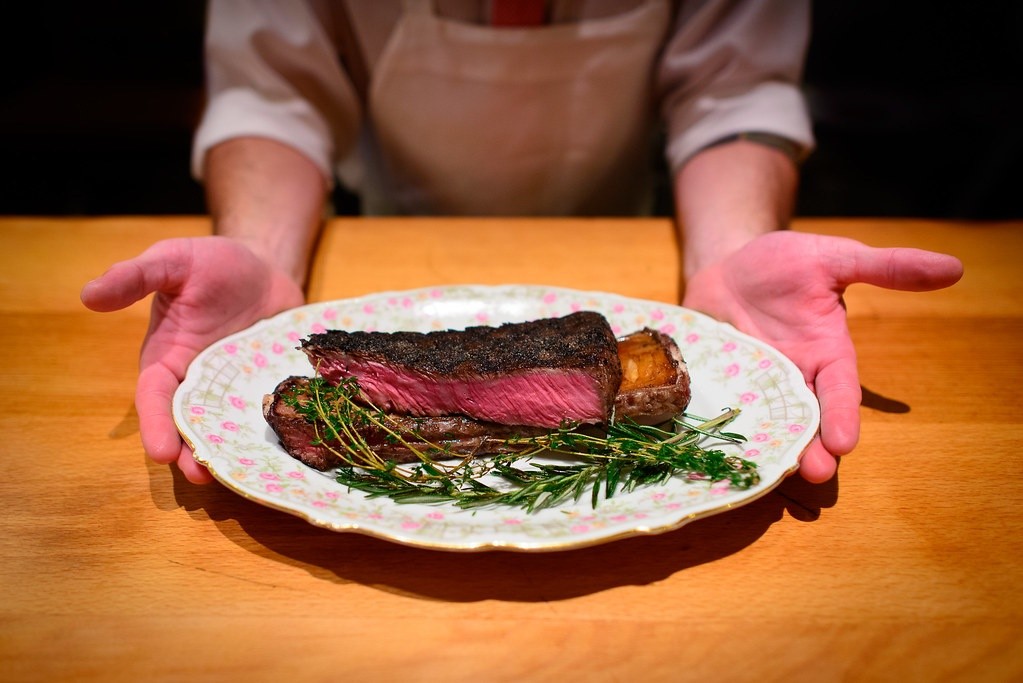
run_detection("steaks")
[295,310,622,426]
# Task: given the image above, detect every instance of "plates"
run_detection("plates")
[171,284,821,554]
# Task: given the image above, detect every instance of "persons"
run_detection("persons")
[79,0,963,484]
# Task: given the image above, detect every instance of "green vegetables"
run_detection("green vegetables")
[280,355,760,516]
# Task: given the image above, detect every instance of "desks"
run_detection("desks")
[1,216,1023,682]
